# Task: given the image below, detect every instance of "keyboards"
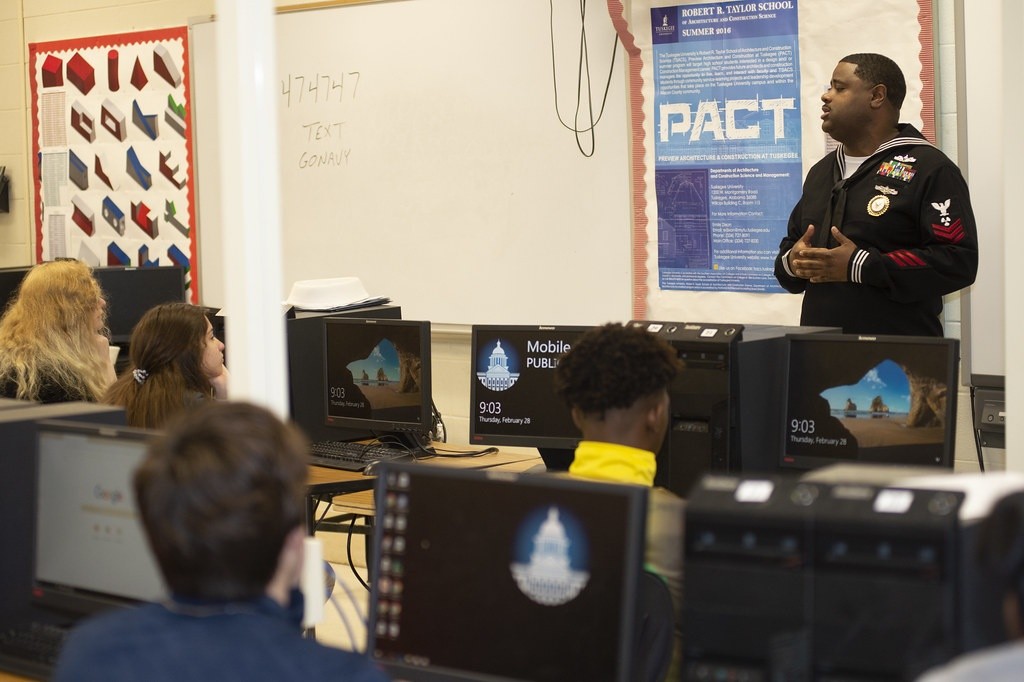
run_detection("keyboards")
[303,440,412,471]
[0,616,76,680]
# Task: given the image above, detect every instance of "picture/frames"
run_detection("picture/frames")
[27,26,202,308]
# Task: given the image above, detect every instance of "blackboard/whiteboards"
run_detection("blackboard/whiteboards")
[186,0,939,345]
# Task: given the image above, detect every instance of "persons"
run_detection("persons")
[914,490,1024,682]
[774,53,978,338]
[1,260,231,429]
[552,323,690,682]
[51,397,394,682]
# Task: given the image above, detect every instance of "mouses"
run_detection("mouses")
[362,460,382,476]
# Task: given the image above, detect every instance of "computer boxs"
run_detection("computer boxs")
[0,395,126,624]
[622,318,1023,682]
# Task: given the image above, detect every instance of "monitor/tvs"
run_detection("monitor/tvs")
[366,462,651,682]
[321,317,434,451]
[92,265,186,351]
[23,421,169,624]
[469,324,599,477]
[781,333,961,471]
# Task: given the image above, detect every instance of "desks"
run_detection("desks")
[303,439,546,653]
